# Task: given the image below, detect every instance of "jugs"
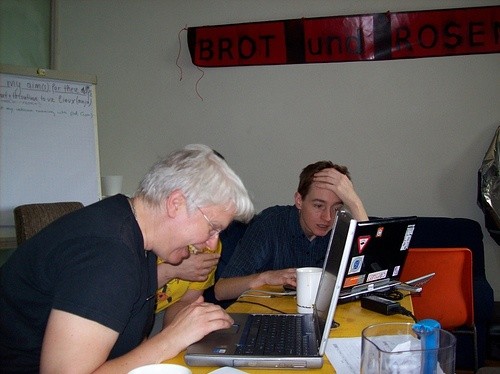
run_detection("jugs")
[359,321,456,374]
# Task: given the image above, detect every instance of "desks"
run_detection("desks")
[162,284,416,374]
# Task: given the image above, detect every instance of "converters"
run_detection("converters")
[360,295,402,316]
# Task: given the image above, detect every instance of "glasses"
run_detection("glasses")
[167,189,225,238]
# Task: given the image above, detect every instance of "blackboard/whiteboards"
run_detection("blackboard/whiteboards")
[0,72,104,249]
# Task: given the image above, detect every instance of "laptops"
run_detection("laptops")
[282,216,417,302]
[183,209,357,369]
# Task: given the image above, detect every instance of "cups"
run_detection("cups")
[295,266,322,314]
[128,363,192,374]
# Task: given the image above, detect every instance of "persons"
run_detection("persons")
[214,161,369,301]
[155,238,221,331]
[0,144,255,374]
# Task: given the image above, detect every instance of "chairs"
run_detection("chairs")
[14,201,85,248]
[400,249,479,374]
